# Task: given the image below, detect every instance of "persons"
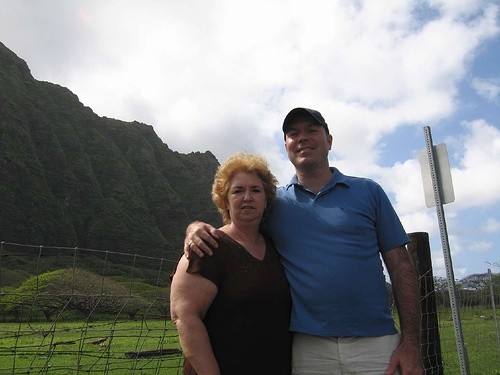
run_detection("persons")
[184,106,425,375]
[168,150,293,375]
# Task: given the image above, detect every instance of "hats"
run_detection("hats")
[283,108,326,140]
